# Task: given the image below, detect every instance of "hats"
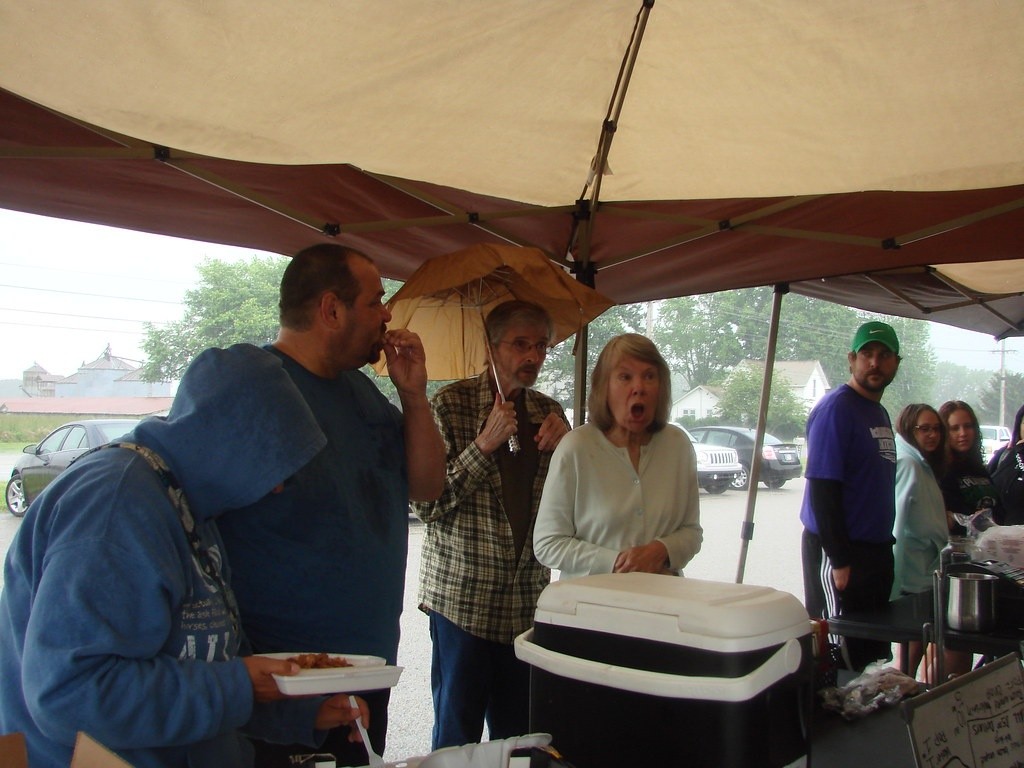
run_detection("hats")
[851,321,900,356]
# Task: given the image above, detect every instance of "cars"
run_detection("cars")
[6,419,141,517]
[685,426,802,491]
[979,425,1011,464]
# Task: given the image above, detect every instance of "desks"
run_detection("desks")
[802,661,931,768]
[827,586,1024,695]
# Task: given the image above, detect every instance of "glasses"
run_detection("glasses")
[913,425,943,434]
[500,341,554,354]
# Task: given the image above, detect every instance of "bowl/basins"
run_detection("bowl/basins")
[945,573,999,631]
[253,653,403,695]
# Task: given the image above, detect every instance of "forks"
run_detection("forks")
[349,696,385,768]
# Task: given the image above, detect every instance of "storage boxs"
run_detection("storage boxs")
[514,571,824,768]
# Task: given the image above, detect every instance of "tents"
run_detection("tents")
[0,0,1024,586]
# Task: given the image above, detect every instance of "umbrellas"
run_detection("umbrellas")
[367,242,616,453]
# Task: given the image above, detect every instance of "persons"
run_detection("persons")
[987,405,1024,527]
[0,344,370,768]
[409,300,573,752]
[800,321,904,676]
[892,403,948,679]
[928,400,999,685]
[533,334,703,582]
[215,243,446,768]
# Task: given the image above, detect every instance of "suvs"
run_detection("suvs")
[667,421,742,493]
